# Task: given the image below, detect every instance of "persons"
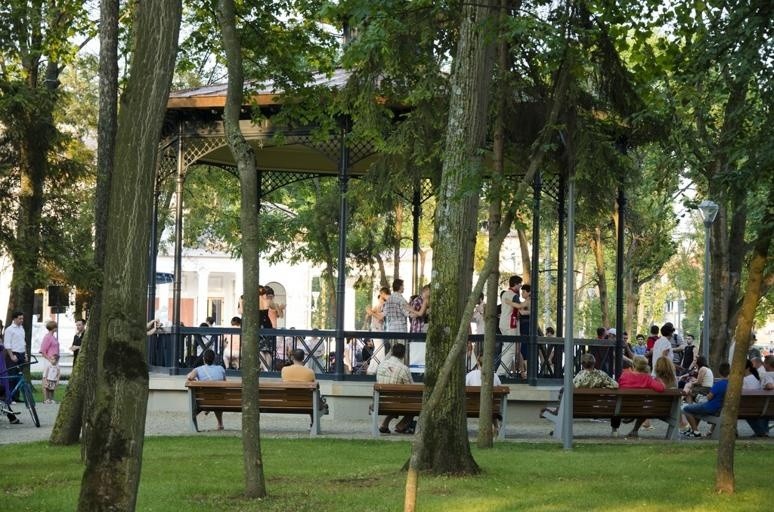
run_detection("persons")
[281,349,328,429]
[0,312,87,424]
[466,355,504,433]
[146,285,374,373]
[568,321,774,438]
[373,343,418,435]
[364,276,535,378]
[186,350,227,430]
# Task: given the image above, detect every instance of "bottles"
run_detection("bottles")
[605,328,617,337]
[695,354,711,370]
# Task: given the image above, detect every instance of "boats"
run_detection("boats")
[49,286,69,306]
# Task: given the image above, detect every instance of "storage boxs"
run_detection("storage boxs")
[697,389,774,442]
[539,386,690,442]
[184,378,331,438]
[367,381,513,442]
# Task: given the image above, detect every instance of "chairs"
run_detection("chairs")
[611,425,712,440]
[379,424,414,433]
[10,420,24,424]
[217,423,225,430]
[45,399,57,404]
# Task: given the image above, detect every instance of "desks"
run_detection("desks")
[697,198,719,369]
